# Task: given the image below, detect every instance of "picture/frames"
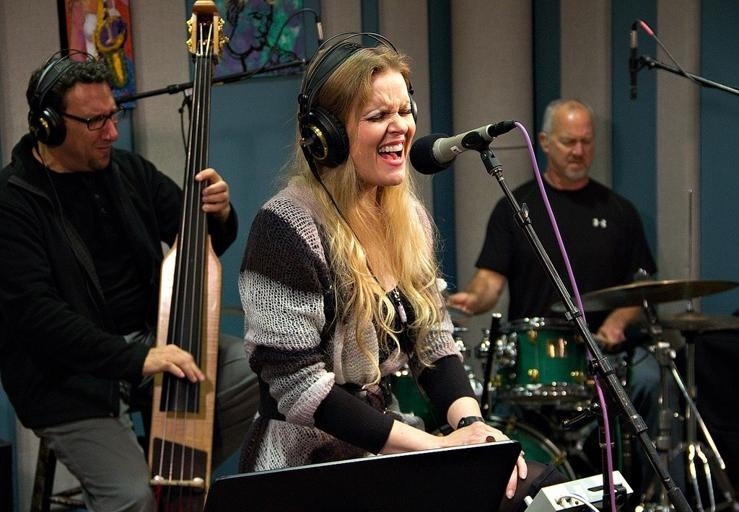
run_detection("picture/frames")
[56,0,136,111]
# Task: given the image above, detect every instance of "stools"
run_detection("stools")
[29,437,89,510]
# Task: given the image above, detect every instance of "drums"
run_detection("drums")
[393,360,477,423]
[429,413,591,493]
[514,315,601,412]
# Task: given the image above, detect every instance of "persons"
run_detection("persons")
[444,99,691,512]
[239,39,594,510]
[0,61,237,511]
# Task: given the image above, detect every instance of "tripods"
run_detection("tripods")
[643,339,739,512]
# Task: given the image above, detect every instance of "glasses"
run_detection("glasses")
[59,98,126,132]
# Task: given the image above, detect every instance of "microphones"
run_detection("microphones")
[629,31,639,101]
[409,120,516,175]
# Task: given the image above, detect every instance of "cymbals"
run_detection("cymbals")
[548,277,738,315]
[627,310,739,336]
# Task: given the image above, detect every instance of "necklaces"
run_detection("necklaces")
[387,277,407,325]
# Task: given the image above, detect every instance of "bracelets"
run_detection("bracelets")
[455,415,487,429]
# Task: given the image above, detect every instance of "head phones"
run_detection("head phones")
[27,49,97,148]
[296,32,418,169]
